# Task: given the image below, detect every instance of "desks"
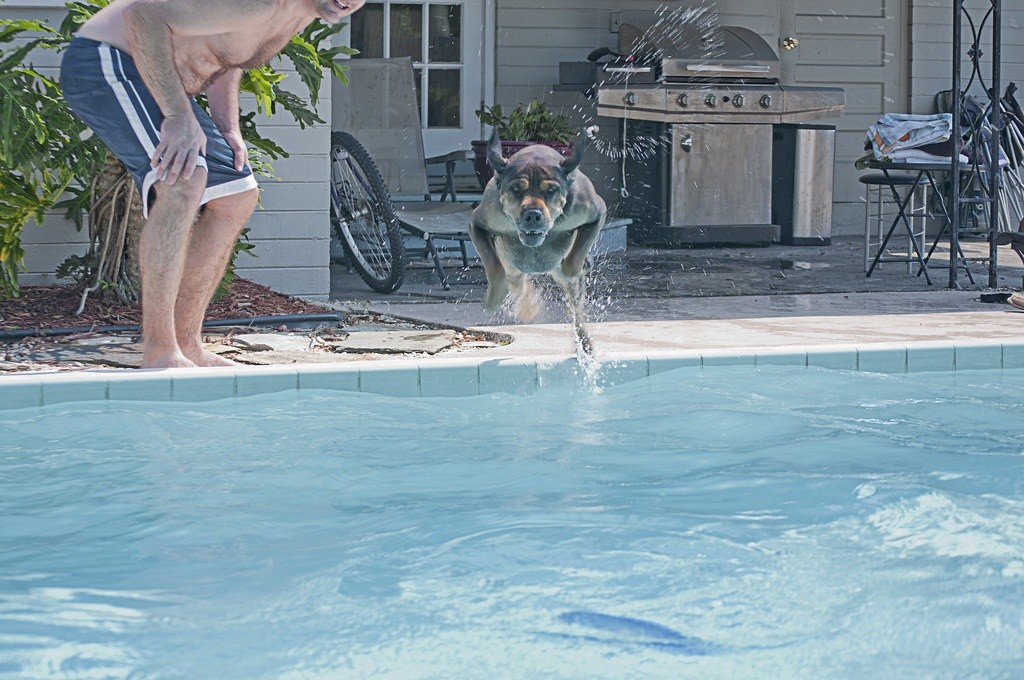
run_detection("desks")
[855,153,1008,285]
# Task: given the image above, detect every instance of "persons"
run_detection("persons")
[59,0,368,369]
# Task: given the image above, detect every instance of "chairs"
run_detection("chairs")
[333,57,633,289]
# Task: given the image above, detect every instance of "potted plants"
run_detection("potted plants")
[472,97,576,192]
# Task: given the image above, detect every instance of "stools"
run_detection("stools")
[859,174,935,275]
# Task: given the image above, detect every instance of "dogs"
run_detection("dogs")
[467,142,608,353]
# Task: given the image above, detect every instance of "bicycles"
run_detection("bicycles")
[330,130,406,295]
